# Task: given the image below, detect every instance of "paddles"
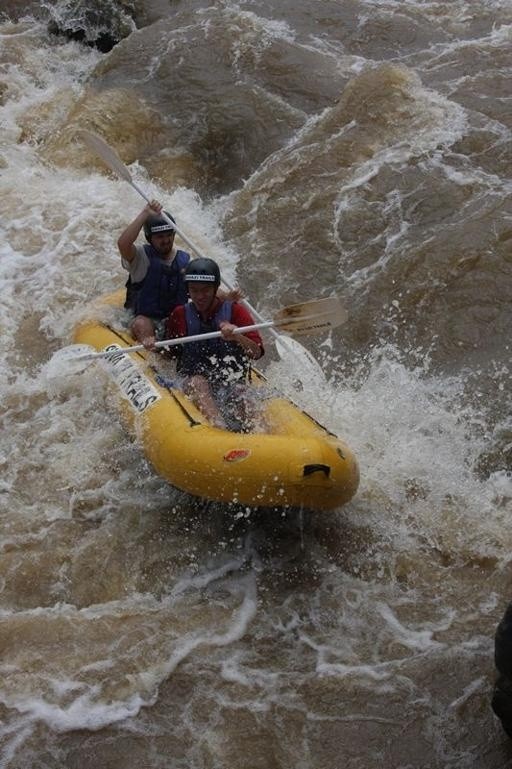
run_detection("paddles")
[76,129,328,382]
[47,297,349,377]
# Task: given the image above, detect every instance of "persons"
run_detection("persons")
[117,198,248,355]
[143,256,266,436]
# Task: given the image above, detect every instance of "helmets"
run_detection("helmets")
[183,257,221,296]
[142,209,176,245]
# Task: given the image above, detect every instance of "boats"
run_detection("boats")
[71,287,360,512]
